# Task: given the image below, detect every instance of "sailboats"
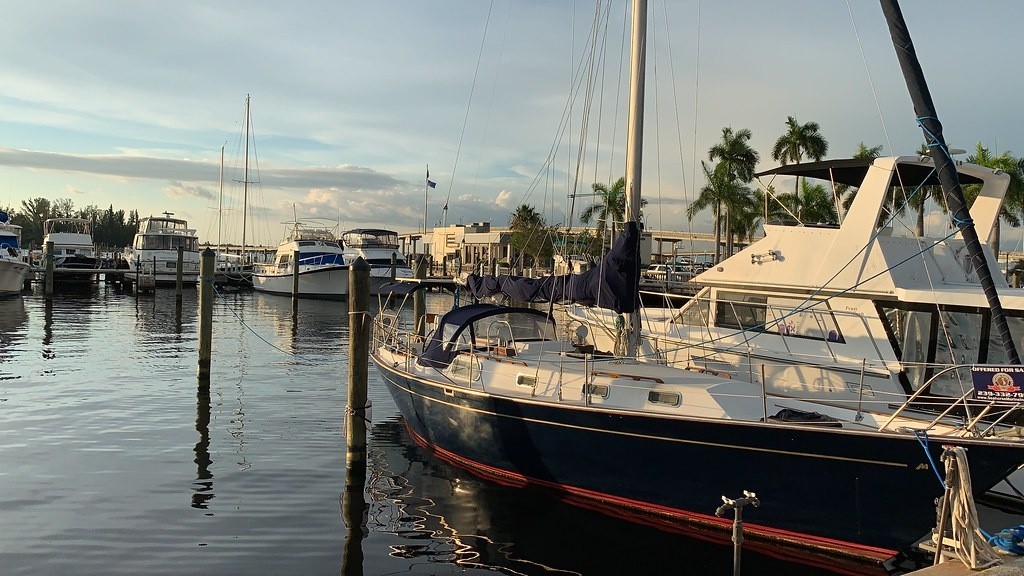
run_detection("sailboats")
[368,0,1024,564]
[207,92,277,274]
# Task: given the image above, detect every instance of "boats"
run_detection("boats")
[122,211,202,286]
[33,205,103,281]
[565,148,1024,509]
[251,199,358,300]
[337,227,416,296]
[0,208,32,297]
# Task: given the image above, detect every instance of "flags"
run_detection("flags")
[428,180,436,188]
[427,168,429,179]
